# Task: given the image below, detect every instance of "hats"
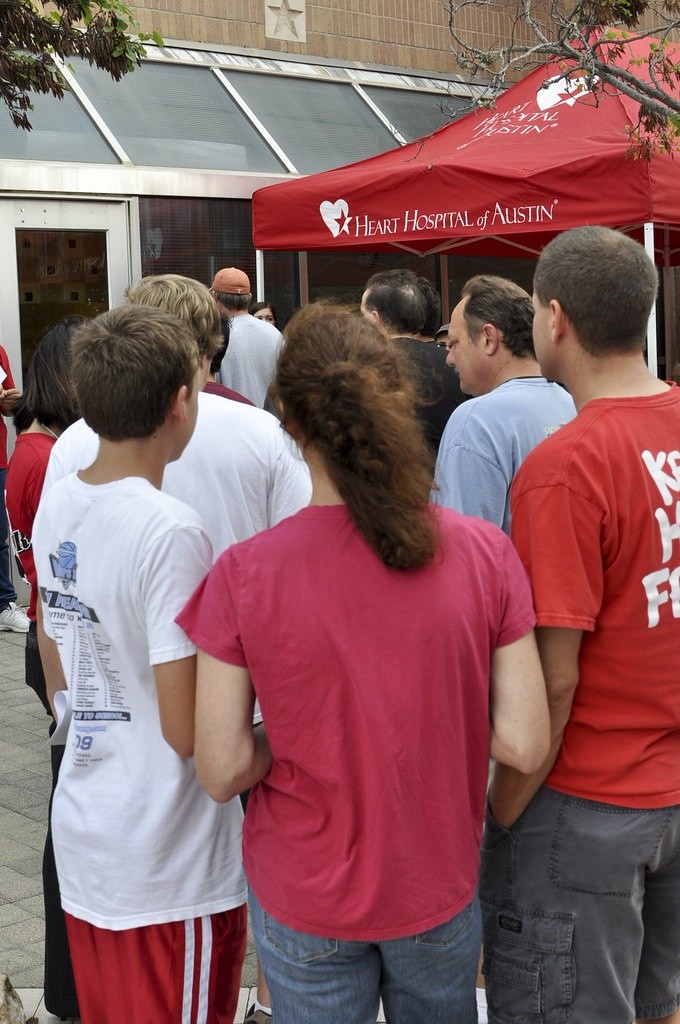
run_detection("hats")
[209,267,250,295]
[433,322,449,335]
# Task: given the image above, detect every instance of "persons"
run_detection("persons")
[174,301,550,1024]
[4,274,313,1024]
[248,302,276,327]
[361,269,579,536]
[0,344,31,633]
[480,225,680,1024]
[208,268,285,409]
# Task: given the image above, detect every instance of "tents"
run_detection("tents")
[252,25,680,378]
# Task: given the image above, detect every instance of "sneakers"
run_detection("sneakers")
[0,601,32,633]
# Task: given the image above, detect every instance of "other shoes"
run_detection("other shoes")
[243,1003,272,1024]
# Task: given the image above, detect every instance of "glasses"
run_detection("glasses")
[435,342,454,349]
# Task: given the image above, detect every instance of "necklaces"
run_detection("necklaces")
[489,376,545,393]
[34,418,58,440]
